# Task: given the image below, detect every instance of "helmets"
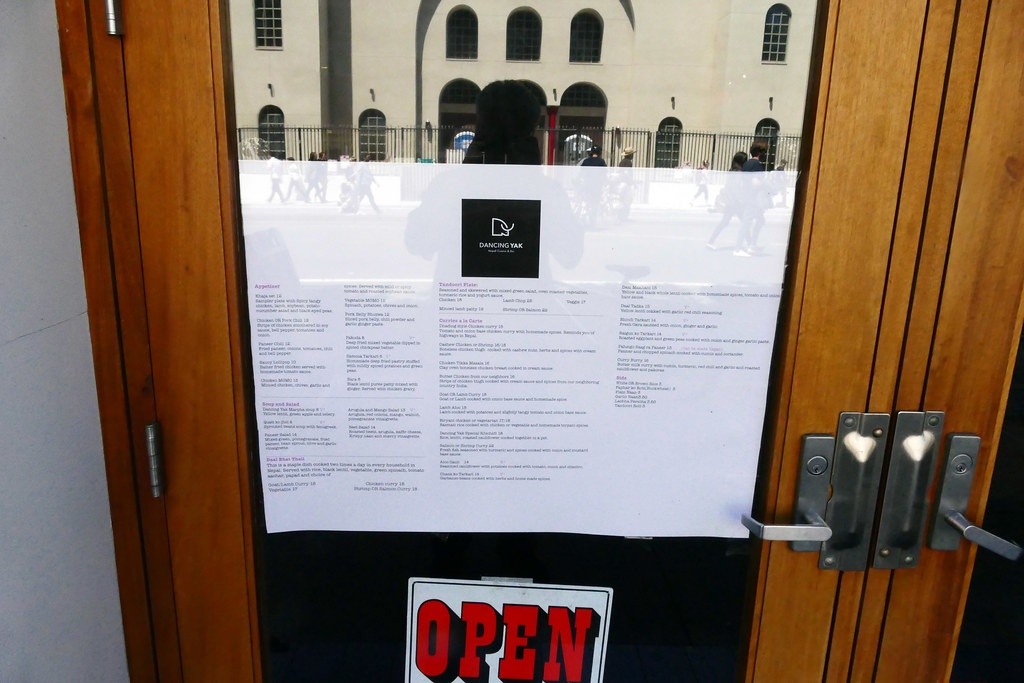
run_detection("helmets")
[591,145,602,154]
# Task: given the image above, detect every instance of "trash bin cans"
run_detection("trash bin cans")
[401,158,442,201]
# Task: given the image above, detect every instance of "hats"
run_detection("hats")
[621,147,637,156]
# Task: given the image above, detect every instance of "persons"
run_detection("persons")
[710,140,771,251]
[261,149,384,214]
[694,161,712,205]
[618,146,638,212]
[461,79,550,166]
[774,158,789,171]
[577,144,607,215]
